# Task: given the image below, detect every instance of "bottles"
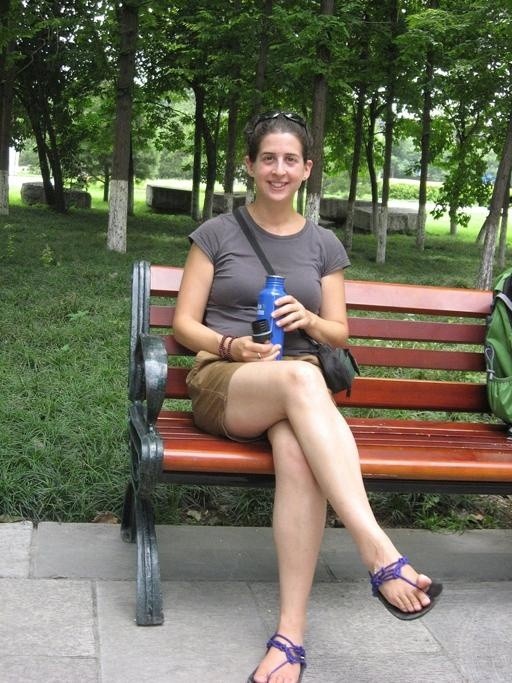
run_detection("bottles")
[251,274,290,362]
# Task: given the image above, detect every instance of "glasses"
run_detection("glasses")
[247,110,310,148]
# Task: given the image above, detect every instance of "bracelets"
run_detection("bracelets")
[219,333,236,362]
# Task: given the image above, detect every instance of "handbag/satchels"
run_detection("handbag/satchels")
[316,343,360,399]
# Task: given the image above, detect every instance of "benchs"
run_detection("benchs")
[119,259,512,628]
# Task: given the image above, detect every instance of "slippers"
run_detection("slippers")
[368,555,443,620]
[247,633,306,682]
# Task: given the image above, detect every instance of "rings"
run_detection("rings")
[257,353,263,359]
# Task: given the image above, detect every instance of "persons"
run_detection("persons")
[173,111,443,683]
